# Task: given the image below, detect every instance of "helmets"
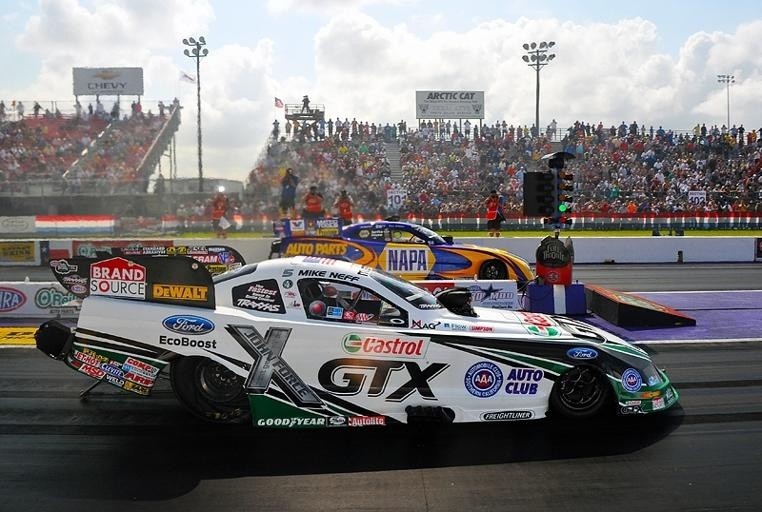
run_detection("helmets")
[391,230,402,239]
[323,286,338,296]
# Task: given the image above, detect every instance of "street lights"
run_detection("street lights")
[715,73,735,126]
[182,36,212,193]
[521,38,555,132]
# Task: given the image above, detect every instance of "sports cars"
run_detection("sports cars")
[267,217,539,289]
[34,252,683,439]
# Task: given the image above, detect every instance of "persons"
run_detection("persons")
[1,95,181,236]
[299,94,313,113]
[562,121,761,231]
[181,117,556,236]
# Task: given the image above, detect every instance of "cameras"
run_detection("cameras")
[288,170,293,176]
[311,191,316,195]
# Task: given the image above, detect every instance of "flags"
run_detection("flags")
[183,71,195,83]
[275,97,284,109]
